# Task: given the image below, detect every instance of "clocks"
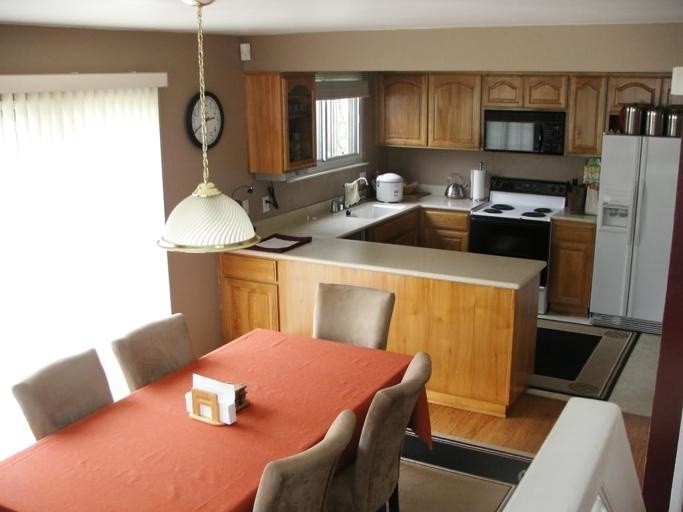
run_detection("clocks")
[182,90,224,150]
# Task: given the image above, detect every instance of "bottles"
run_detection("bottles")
[332,200,338,212]
[291,132,306,162]
[619,104,683,138]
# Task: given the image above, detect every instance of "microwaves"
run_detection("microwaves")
[482,109,566,156]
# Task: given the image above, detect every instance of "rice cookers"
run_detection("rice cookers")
[375,173,405,204]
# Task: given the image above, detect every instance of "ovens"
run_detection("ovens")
[468,216,549,315]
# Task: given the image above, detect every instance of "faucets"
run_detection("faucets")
[342,177,369,188]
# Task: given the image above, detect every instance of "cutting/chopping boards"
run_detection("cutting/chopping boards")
[403,191,430,199]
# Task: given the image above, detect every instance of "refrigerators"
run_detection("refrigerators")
[587,130,682,337]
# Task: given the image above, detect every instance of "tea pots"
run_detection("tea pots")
[443,173,469,199]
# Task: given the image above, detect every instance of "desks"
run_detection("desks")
[0,322,427,512]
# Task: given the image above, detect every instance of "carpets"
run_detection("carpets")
[384,416,537,512]
[530,309,645,402]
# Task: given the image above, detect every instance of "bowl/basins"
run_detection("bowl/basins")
[403,182,417,194]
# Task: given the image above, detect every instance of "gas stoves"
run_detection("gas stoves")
[470,202,561,221]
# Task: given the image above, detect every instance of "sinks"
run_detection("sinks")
[333,201,409,221]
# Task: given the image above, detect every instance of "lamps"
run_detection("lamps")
[668,65,682,96]
[226,183,256,199]
[157,0,262,258]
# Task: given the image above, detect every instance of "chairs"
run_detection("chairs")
[251,407,357,512]
[332,345,432,512]
[309,280,399,350]
[112,309,196,391]
[10,343,113,443]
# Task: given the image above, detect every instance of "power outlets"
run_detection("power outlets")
[262,194,272,215]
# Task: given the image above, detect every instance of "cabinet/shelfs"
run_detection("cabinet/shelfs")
[242,71,317,178]
[367,71,427,148]
[421,203,469,254]
[546,217,595,320]
[480,70,567,112]
[211,250,280,340]
[563,71,606,157]
[604,72,682,115]
[363,205,425,245]
[426,71,483,152]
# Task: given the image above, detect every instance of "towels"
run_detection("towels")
[344,180,360,209]
